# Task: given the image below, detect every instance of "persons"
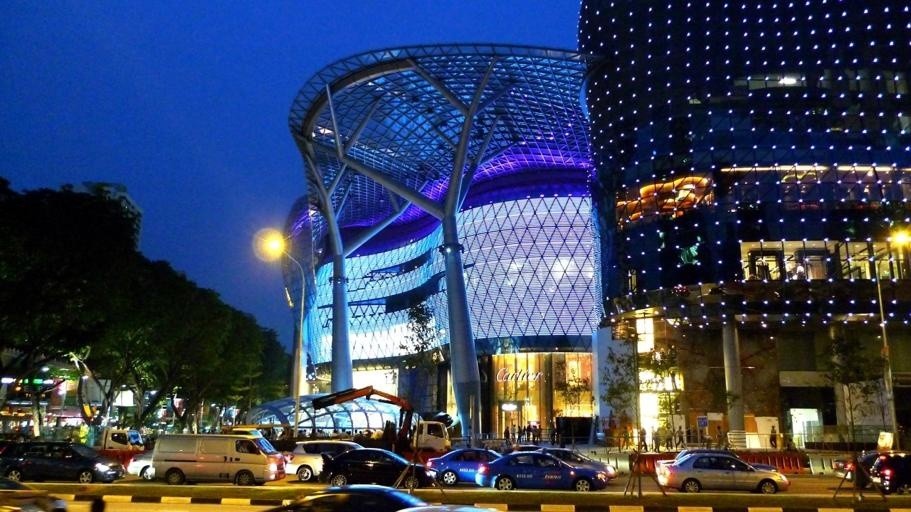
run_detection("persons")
[468,426,473,444]
[770,426,777,448]
[265,426,362,440]
[540,459,549,466]
[701,436,713,449]
[622,426,629,449]
[653,427,661,453]
[788,426,792,451]
[716,426,723,450]
[675,426,685,448]
[638,428,648,451]
[665,426,673,449]
[239,440,250,453]
[504,425,543,445]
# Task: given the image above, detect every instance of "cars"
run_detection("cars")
[655,449,779,475]
[127,449,157,480]
[832,451,911,494]
[656,453,791,495]
[476,451,609,491]
[390,502,499,511]
[507,444,618,482]
[254,481,430,511]
[0,477,69,511]
[426,448,503,488]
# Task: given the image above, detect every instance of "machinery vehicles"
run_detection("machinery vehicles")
[311,384,456,452]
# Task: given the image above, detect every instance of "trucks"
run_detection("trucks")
[231,427,264,437]
[89,425,146,452]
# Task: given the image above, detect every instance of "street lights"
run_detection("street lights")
[252,226,308,439]
[873,225,911,451]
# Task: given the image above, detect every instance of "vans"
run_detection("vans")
[151,432,288,487]
[283,440,367,480]
[0,441,126,484]
